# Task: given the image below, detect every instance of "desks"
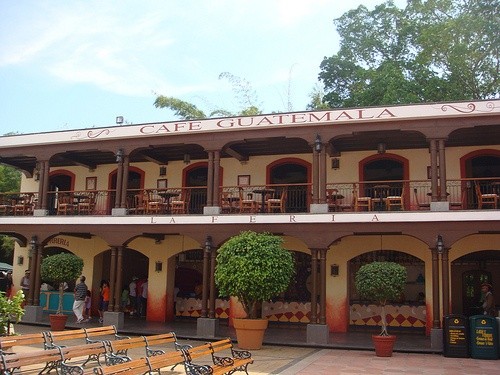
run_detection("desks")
[158,193,179,213]
[71,195,88,215]
[372,185,390,211]
[253,189,275,213]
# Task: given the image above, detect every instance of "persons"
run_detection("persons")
[5,271,14,301]
[98,276,148,323]
[20,270,32,305]
[73,275,92,324]
[480,282,497,317]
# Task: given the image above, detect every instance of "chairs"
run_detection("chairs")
[135,193,191,214]
[0,196,32,216]
[220,187,288,213]
[474,180,497,209]
[326,184,406,211]
[55,193,96,215]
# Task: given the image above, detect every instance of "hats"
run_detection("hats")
[131,276,137,281]
[480,282,493,289]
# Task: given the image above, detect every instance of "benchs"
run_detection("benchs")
[0,325,254,375]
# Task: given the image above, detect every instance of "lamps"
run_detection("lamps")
[29,234,37,248]
[436,235,445,255]
[205,237,212,256]
[115,148,124,165]
[313,134,385,153]
[160,153,190,176]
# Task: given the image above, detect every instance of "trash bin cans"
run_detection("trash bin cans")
[469,315,498,359]
[444,317,468,357]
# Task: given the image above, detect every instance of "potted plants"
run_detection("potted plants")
[355,261,408,357]
[214,229,297,349]
[40,252,84,331]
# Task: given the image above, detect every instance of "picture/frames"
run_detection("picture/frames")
[86,177,96,190]
[157,179,167,188]
[427,166,440,179]
[238,175,250,184]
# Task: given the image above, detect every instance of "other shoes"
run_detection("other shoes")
[76,317,84,325]
[98,318,103,323]
[83,316,93,323]
